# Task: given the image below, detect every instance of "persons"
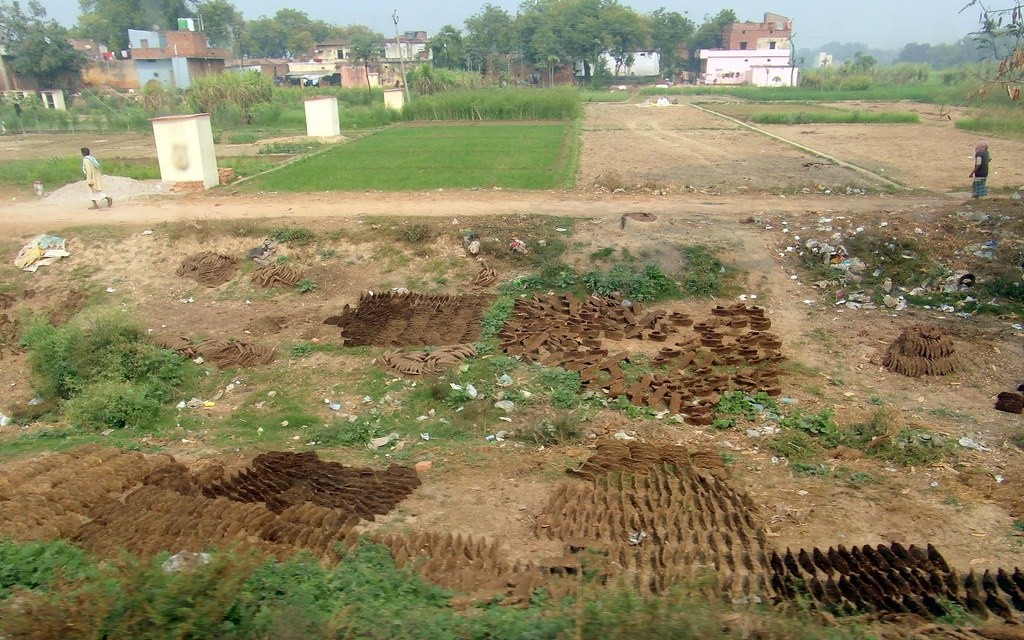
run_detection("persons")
[80,148,112,210]
[968,141,992,200]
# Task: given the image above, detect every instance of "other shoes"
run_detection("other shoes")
[108,198,112,207]
[88,206,99,210]
